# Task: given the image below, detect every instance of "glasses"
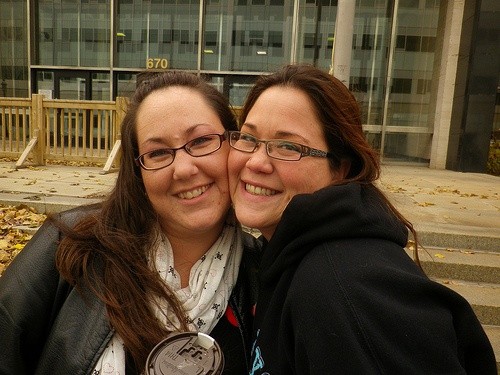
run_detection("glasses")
[134,130,233,171]
[228,128,346,162]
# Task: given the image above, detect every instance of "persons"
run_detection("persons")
[226,64,498,375]
[0,70,263,375]
